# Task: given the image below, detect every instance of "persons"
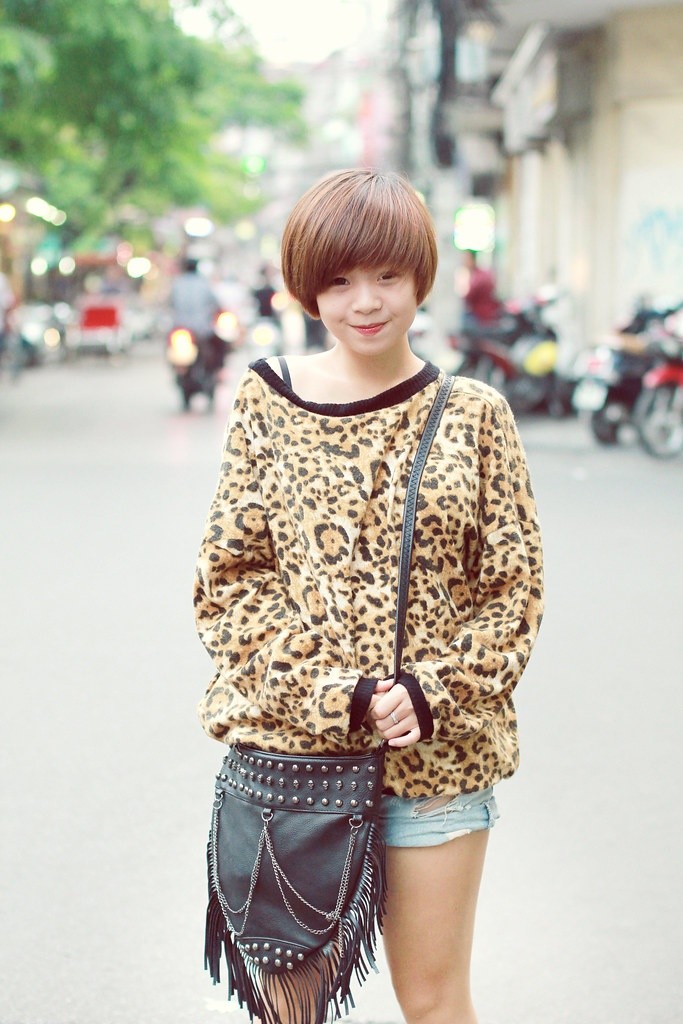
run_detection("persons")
[171,255,225,375]
[453,248,502,377]
[245,265,281,327]
[192,169,546,1024]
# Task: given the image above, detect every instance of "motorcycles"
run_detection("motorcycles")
[591,299,683,456]
[177,281,586,424]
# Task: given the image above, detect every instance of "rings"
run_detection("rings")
[390,713,398,725]
